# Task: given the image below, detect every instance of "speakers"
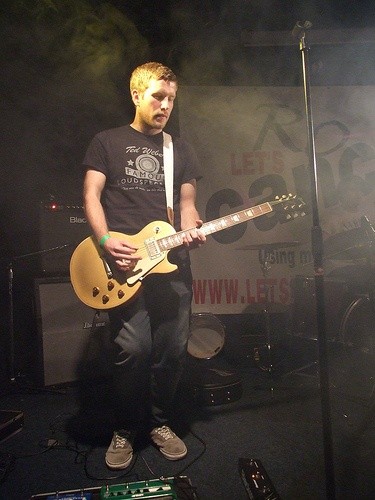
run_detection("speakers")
[14,275,115,394]
[30,203,94,276]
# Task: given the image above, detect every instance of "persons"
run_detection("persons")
[84,61,207,467]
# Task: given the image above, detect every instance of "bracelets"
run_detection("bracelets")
[99,234,113,251]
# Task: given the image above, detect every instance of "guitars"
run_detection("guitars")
[70,191,307,313]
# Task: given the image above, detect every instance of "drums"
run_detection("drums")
[336,295,375,373]
[186,315,229,362]
[296,274,367,343]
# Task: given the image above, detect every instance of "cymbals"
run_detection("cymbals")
[238,240,302,251]
[321,222,374,261]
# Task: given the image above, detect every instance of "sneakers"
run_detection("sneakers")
[147,424,187,461]
[105,428,135,470]
